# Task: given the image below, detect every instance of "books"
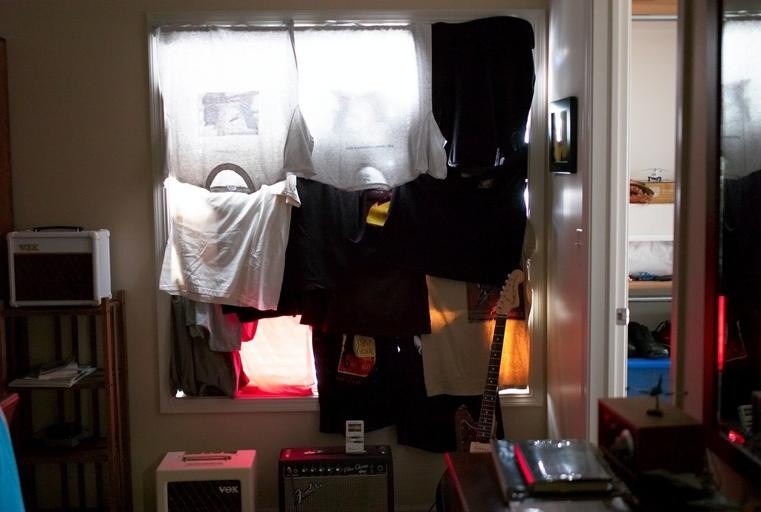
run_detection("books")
[628,358,670,395]
[278,444,395,511]
[631,179,675,203]
[156,450,258,512]
[8,226,111,305]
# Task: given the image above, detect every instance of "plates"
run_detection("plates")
[0,289,132,512]
[626,203,674,401]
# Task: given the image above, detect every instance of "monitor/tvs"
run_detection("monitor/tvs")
[9,362,95,388]
[515,438,614,494]
[40,357,78,380]
[489,437,630,502]
[8,365,90,382]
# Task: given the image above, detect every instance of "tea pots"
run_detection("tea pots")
[702,12,761,477]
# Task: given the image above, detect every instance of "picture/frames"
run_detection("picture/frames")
[444,447,761,512]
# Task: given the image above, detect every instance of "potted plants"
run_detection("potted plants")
[548,95,578,174]
